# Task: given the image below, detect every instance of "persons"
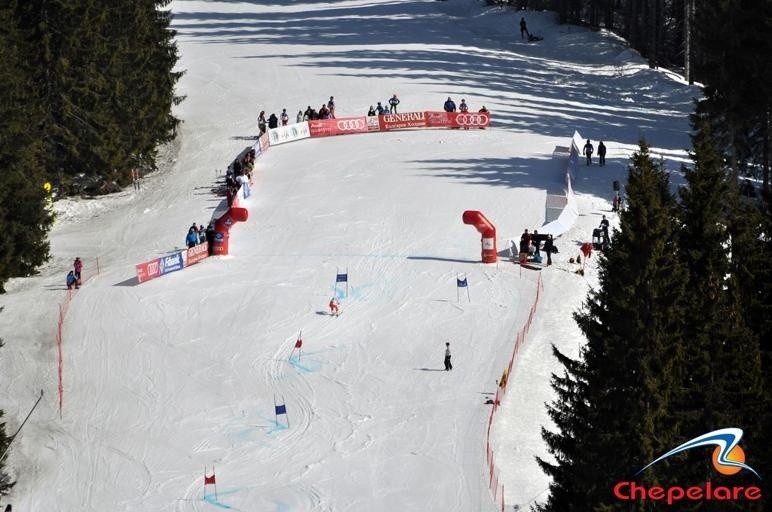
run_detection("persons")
[185,92,495,250]
[68,271,80,290]
[597,140,607,167]
[519,229,554,266]
[73,257,83,279]
[444,342,454,371]
[586,140,594,166]
[329,296,341,315]
[598,215,611,245]
[520,16,530,39]
